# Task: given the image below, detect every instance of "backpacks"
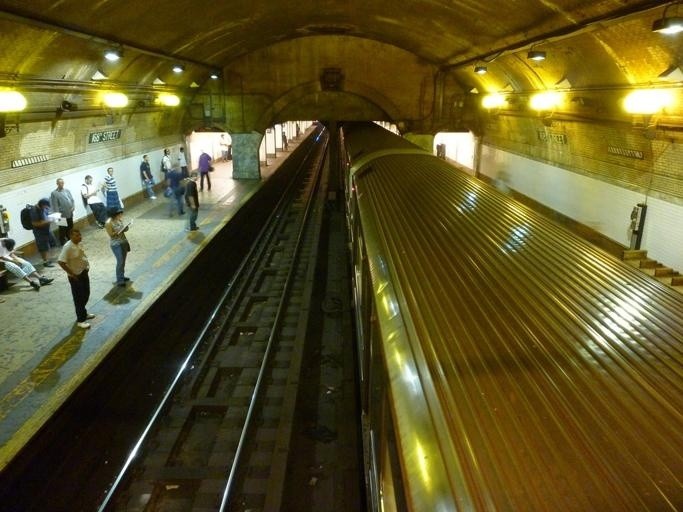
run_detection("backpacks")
[20,203,36,230]
[81,184,89,205]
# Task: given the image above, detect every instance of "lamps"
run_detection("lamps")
[103,36,126,62]
[528,41,548,60]
[651,1,683,36]
[474,57,488,74]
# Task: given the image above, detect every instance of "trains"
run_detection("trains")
[342,120,683,512]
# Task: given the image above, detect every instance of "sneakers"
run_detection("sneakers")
[39,275,55,286]
[30,280,40,293]
[86,313,95,319]
[76,321,90,329]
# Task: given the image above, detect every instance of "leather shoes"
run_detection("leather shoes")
[117,283,126,288]
[124,277,130,281]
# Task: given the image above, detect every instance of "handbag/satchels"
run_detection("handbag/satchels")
[176,186,184,196]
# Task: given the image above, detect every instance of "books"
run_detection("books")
[117,219,132,235]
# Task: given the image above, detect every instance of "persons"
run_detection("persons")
[105,206,130,287]
[220,134,229,162]
[51,178,75,245]
[282,132,288,148]
[81,175,107,229]
[104,167,122,210]
[58,228,94,328]
[0,237,54,290]
[140,146,213,232]
[296,124,300,137]
[29,198,54,267]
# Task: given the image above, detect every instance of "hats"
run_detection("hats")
[110,206,124,216]
[40,198,51,208]
[6,239,15,251]
